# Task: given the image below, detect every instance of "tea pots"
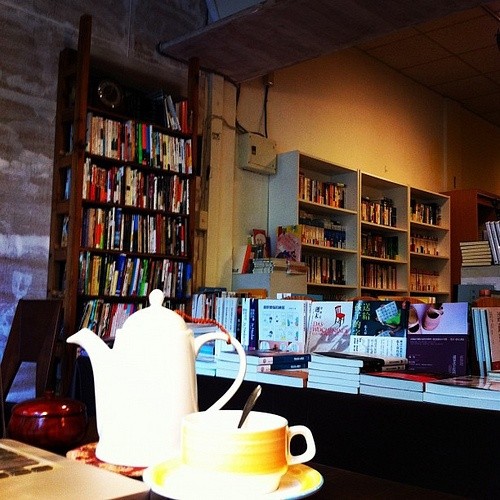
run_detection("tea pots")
[66,289,246,468]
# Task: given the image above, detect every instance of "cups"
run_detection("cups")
[181,410,316,493]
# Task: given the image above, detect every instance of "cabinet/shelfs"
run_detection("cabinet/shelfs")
[46,14,199,399]
[360,169,451,302]
[267,150,360,300]
[438,190,500,286]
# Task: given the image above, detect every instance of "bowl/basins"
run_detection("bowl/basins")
[8,390,89,448]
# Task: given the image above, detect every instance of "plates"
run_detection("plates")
[143,456,325,500]
